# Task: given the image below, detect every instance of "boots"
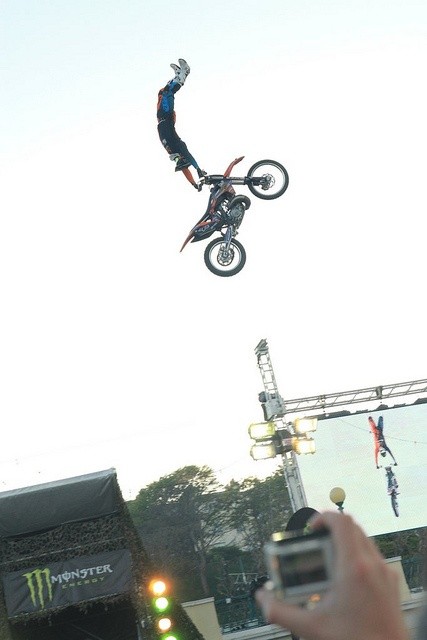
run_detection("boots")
[170,64,180,82]
[174,58,190,86]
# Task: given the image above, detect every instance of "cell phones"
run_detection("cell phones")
[264,527,335,639]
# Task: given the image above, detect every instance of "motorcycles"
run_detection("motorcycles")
[180,155,290,278]
[377,462,400,518]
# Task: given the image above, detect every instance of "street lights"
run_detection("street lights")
[329,485,347,517]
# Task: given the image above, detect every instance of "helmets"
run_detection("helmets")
[169,152,191,172]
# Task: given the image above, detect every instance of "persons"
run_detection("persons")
[156,59,203,189]
[367,415,397,469]
[254,512,411,640]
